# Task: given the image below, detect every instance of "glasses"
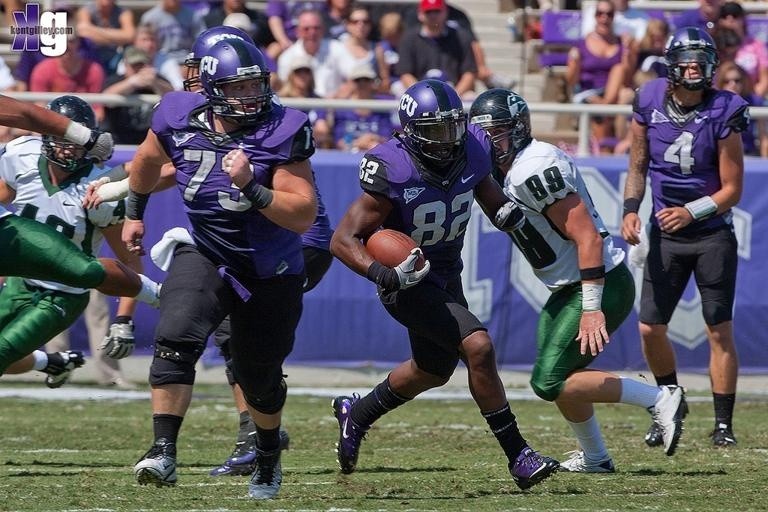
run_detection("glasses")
[594,10,614,18]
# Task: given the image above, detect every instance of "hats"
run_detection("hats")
[350,63,377,82]
[287,58,312,75]
[122,47,149,67]
[418,0,446,14]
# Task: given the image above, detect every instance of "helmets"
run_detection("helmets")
[467,86,533,166]
[197,37,274,127]
[192,25,256,62]
[398,79,468,176]
[664,26,720,91]
[40,95,97,170]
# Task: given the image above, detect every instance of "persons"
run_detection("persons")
[468,87,686,471]
[329,81,559,490]
[0,0,768,156]
[120,39,319,498]
[45,153,138,390]
[0,94,165,308]
[621,26,750,448]
[82,27,335,476]
[0,96,143,388]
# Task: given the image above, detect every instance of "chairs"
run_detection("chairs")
[540,9,768,121]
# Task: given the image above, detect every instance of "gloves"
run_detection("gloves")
[96,318,137,360]
[87,127,114,164]
[383,247,431,297]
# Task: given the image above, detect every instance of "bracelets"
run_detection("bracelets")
[685,196,717,223]
[582,284,604,311]
[125,188,151,220]
[368,261,388,283]
[623,198,640,218]
[65,119,91,145]
[581,266,604,281]
[239,180,273,209]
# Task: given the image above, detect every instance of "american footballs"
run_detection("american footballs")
[365,227,425,271]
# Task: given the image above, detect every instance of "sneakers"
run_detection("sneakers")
[247,434,283,500]
[43,347,86,389]
[224,425,291,471]
[649,384,687,455]
[713,416,737,448]
[507,444,562,491]
[558,451,615,474]
[644,398,689,446]
[133,437,179,487]
[330,393,373,473]
[208,440,255,477]
